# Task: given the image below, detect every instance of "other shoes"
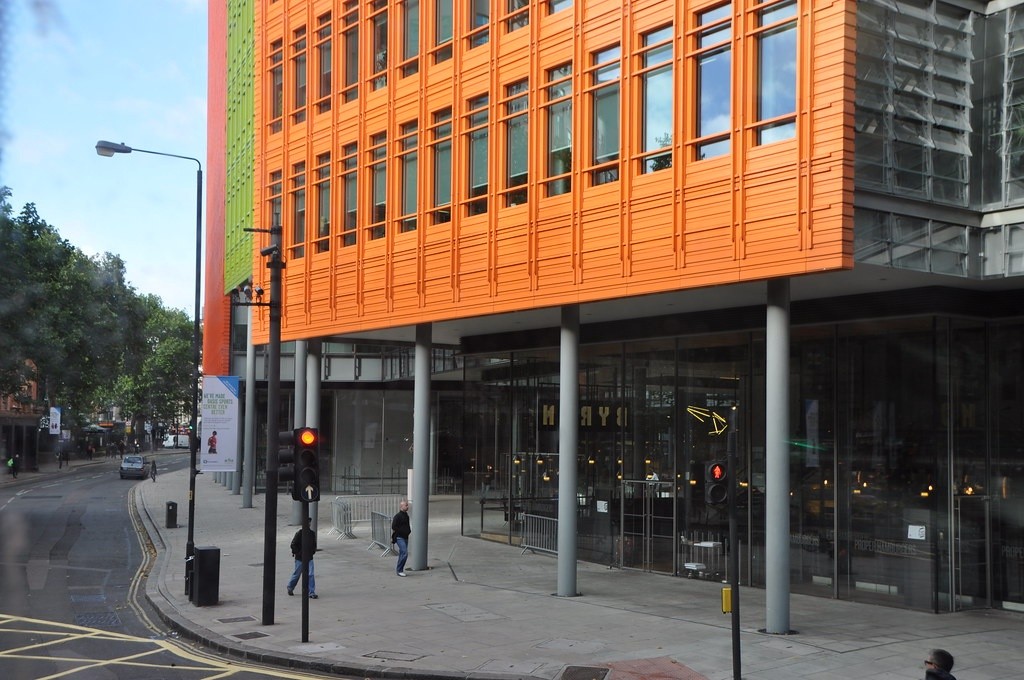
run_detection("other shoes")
[286,586,293,595]
[397,572,407,577]
[309,593,318,599]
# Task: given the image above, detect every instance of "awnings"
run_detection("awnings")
[100,422,113,426]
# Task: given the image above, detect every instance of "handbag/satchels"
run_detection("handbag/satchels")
[391,531,397,543]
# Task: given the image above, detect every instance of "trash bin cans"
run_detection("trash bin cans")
[166,501,177,527]
[193,546,220,607]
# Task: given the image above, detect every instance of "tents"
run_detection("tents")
[81,424,111,447]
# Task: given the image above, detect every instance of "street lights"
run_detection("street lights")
[94,140,204,599]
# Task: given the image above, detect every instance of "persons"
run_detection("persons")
[56,453,64,470]
[13,454,19,477]
[392,502,411,577]
[208,431,218,454]
[105,439,125,459]
[287,517,318,599]
[86,444,95,461]
[150,460,156,482]
[925,649,956,680]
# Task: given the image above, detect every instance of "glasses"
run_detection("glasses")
[925,660,937,666]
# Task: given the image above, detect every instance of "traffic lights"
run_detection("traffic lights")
[704,459,728,507]
[292,427,320,503]
[278,429,299,484]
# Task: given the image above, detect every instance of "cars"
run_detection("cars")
[120,455,150,480]
